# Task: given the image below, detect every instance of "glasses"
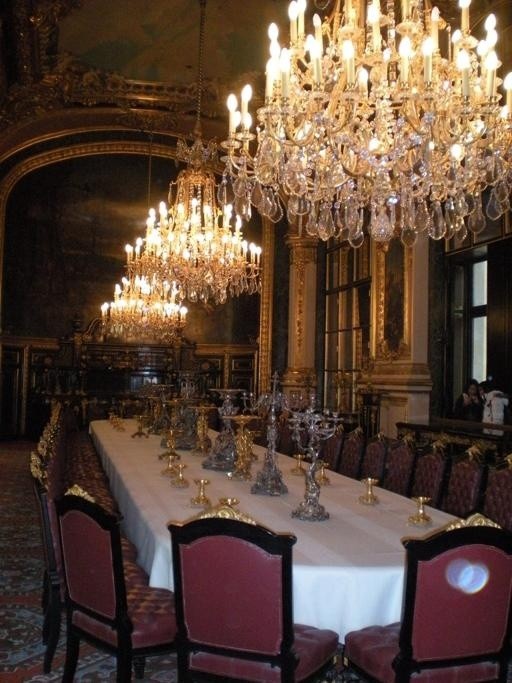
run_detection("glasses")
[221,0,512,247]
[124,120,261,304]
[100,277,188,346]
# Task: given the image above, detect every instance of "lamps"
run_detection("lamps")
[167,505,319,682]
[344,513,512,682]
[30,466,149,651]
[355,431,388,485]
[379,431,417,497]
[338,426,364,479]
[491,454,512,533]
[37,400,101,464]
[444,445,487,521]
[280,419,293,458]
[55,485,179,683]
[408,441,449,510]
[31,450,138,606]
[325,425,341,472]
